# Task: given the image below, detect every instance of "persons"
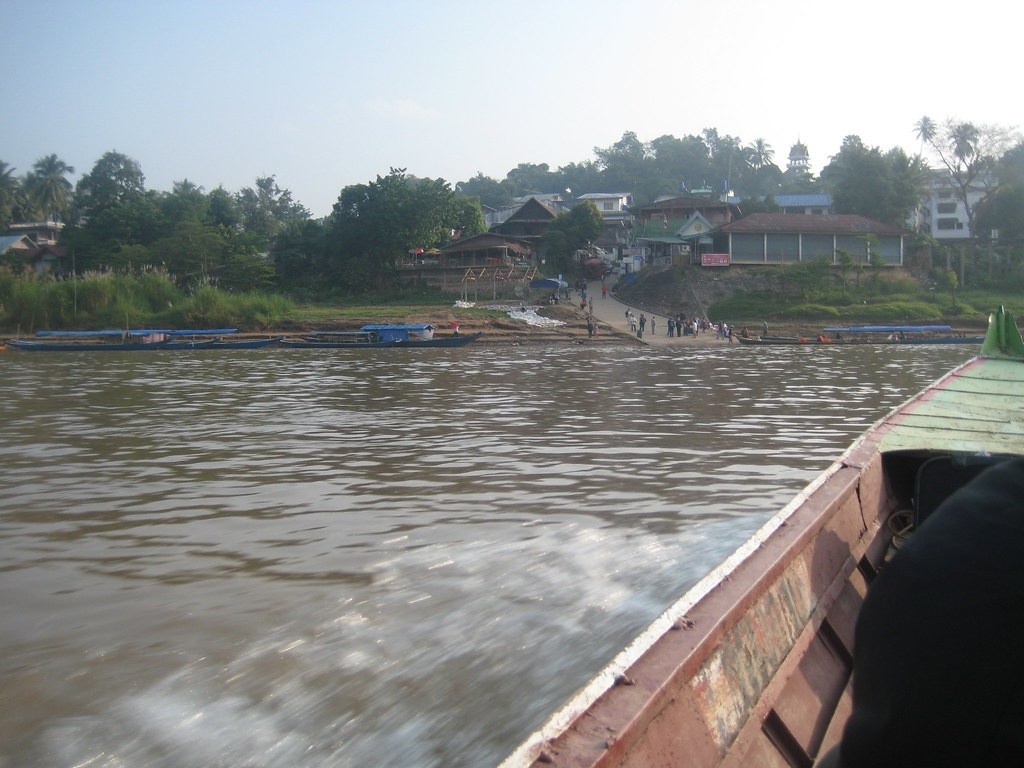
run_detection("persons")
[580,281,594,313]
[602,285,607,299]
[718,322,735,344]
[626,308,706,338]
[549,294,559,305]
[763,322,768,336]
[817,334,824,342]
[585,314,599,337]
[453,325,459,336]
[834,333,841,339]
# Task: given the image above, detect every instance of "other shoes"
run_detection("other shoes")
[716,337,719,339]
[722,338,724,340]
[677,335,681,337]
[666,333,669,335]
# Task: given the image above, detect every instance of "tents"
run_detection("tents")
[529,278,568,289]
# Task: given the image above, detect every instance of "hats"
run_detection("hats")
[731,325,734,327]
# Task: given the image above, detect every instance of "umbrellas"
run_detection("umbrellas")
[585,258,600,266]
[410,248,440,255]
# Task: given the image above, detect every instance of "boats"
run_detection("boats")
[731,323,986,345]
[279,324,484,348]
[1,329,286,350]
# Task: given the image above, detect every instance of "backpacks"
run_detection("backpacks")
[726,330,730,336]
